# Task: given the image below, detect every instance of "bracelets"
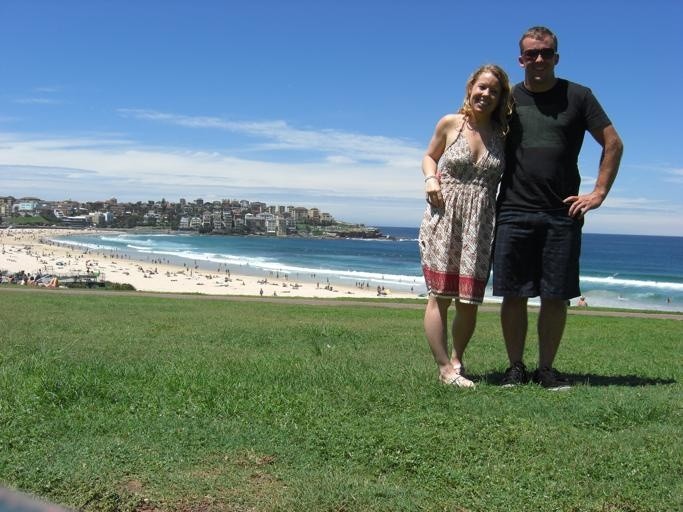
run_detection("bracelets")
[423,174,437,183]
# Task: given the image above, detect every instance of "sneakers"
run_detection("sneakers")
[450,359,464,374]
[439,373,474,389]
[535,367,573,386]
[501,363,527,386]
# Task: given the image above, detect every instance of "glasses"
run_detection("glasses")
[524,48,555,60]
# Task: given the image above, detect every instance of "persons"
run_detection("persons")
[490,24,623,394]
[0,227,416,297]
[416,61,514,391]
[574,294,588,308]
